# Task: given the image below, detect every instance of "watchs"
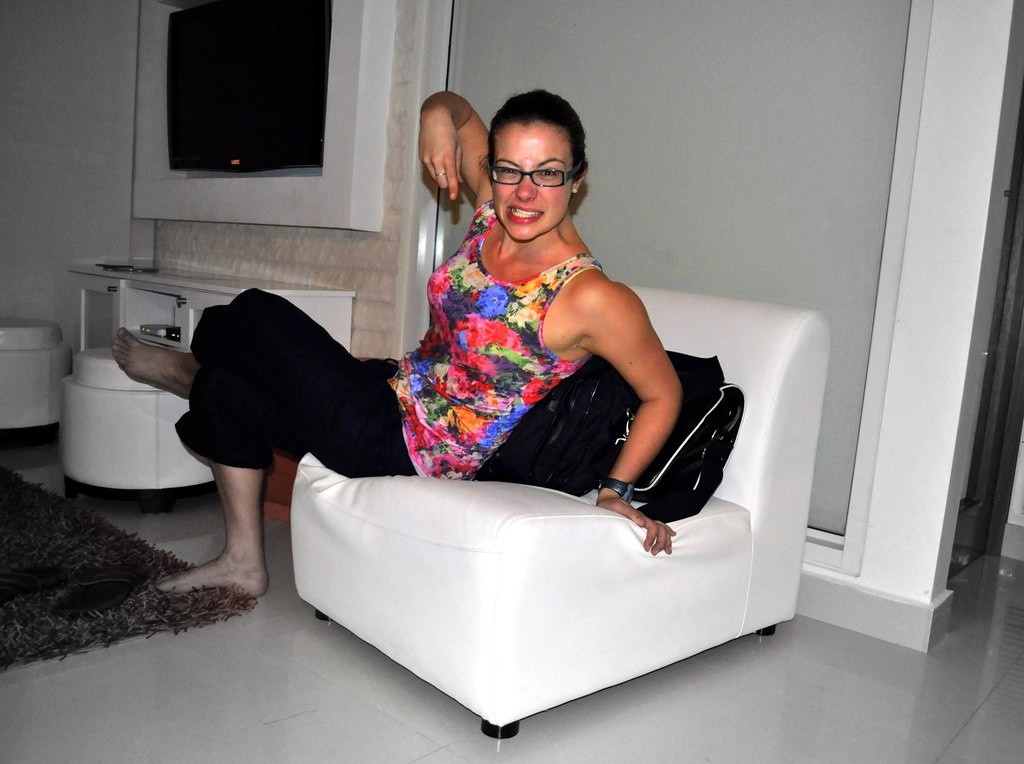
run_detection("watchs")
[598,478,634,504]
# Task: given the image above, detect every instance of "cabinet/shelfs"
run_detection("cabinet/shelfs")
[68,261,357,355]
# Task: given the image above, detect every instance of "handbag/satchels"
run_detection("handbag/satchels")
[473,349,743,523]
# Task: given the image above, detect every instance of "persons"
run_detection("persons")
[112,91,686,596]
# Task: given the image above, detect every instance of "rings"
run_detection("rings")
[436,173,446,176]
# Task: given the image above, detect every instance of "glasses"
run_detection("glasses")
[485,158,582,187]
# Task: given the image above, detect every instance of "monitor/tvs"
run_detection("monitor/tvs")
[167,0,332,174]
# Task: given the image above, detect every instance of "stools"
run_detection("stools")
[62,345,235,499]
[0,319,68,449]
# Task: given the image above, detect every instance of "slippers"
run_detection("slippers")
[0,565,67,606]
[62,561,133,612]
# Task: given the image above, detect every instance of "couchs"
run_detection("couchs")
[288,279,835,740]
